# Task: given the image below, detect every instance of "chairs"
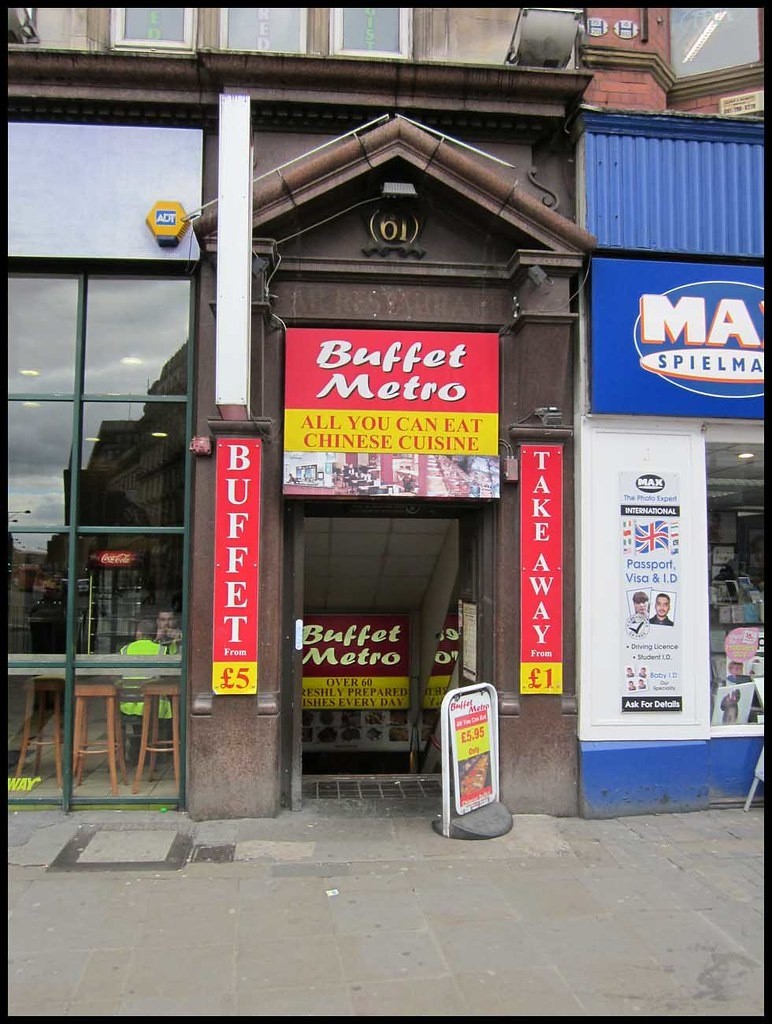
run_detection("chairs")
[109,675,168,773]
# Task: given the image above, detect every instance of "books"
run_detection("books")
[709,576,763,623]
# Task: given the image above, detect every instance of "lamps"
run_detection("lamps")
[380,182,417,200]
[506,7,585,70]
[152,381,169,437]
[253,257,270,277]
[527,266,548,287]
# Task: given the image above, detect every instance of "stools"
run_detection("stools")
[13,675,181,797]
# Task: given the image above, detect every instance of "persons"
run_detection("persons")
[633,591,649,615]
[649,594,673,625]
[714,560,737,581]
[8,580,68,765]
[750,535,764,567]
[728,662,744,678]
[117,609,182,754]
[626,667,646,691]
